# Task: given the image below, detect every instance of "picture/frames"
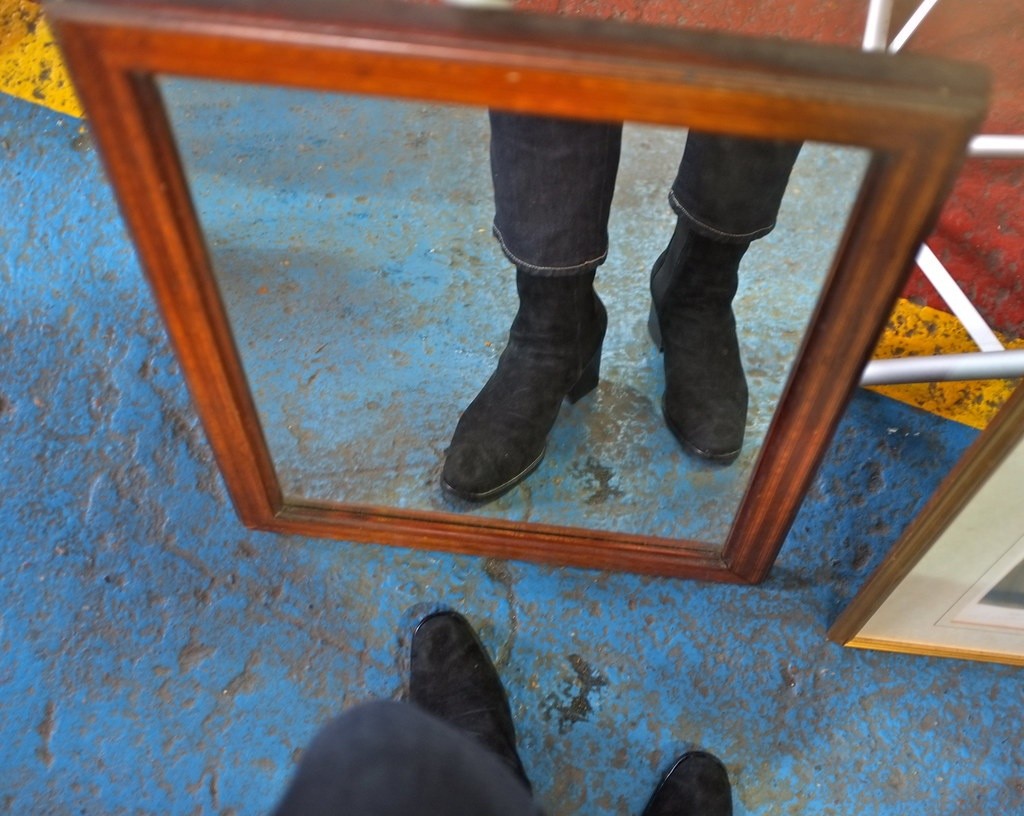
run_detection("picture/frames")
[829,373,1024,666]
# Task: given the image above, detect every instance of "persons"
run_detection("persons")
[440,107,804,507]
[270,611,735,816]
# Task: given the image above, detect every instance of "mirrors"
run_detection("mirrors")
[41,1,991,584]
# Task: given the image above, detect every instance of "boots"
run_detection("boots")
[640,750,734,816]
[648,215,748,463]
[439,264,608,501]
[408,610,533,797]
